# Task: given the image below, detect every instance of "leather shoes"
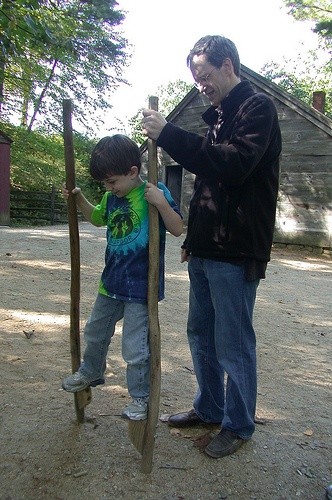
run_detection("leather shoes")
[168,409,205,428]
[205,428,245,457]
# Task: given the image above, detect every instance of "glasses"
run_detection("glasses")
[193,66,217,89]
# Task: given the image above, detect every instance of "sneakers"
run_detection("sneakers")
[62,370,105,393]
[122,396,148,421]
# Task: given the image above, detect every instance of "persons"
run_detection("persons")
[62,134,183,421]
[143,35,282,458]
